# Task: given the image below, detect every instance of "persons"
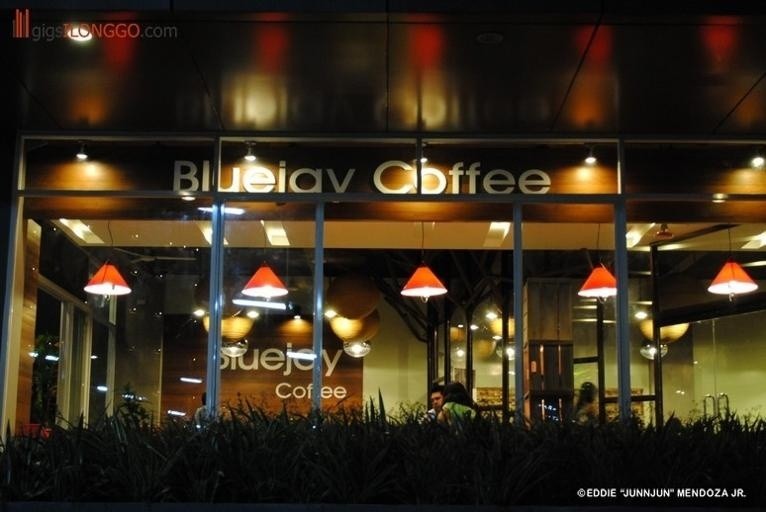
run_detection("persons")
[419,385,445,424]
[437,381,479,425]
[194,393,206,417]
[572,381,598,417]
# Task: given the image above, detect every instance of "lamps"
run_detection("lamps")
[83,246,133,302]
[400,221,448,302]
[577,220,618,304]
[240,221,288,303]
[707,221,759,304]
